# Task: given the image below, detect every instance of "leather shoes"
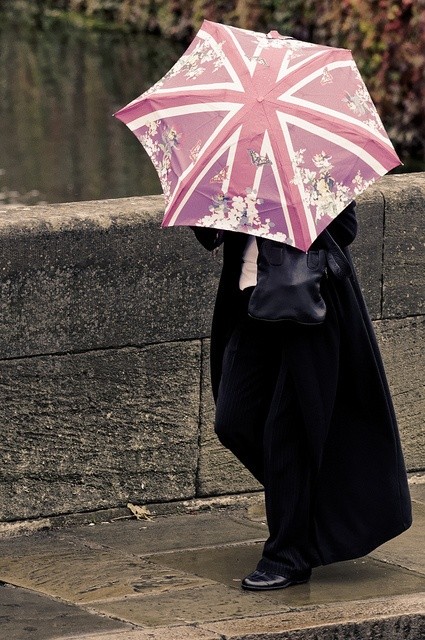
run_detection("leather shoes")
[239,570,309,589]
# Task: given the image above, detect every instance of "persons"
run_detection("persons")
[188,196,414,591]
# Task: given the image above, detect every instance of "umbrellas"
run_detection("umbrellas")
[110,16,407,254]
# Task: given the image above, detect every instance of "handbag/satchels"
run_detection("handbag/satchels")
[248,232,353,322]
